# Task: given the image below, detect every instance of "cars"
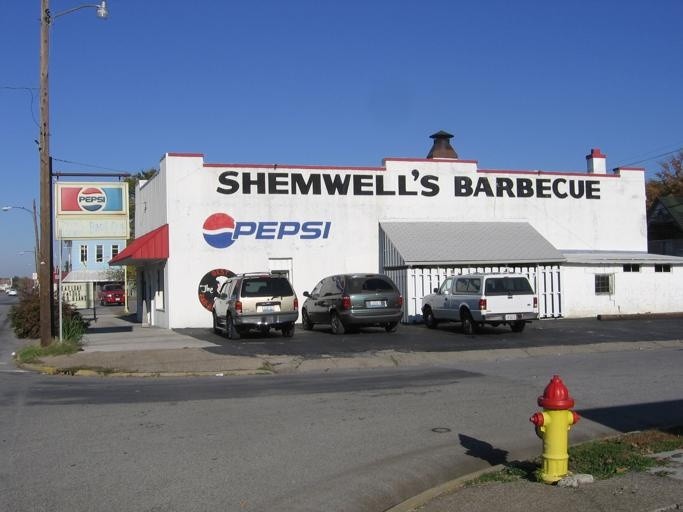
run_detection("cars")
[421,273,539,334]
[210,273,298,340]
[100,284,124,305]
[302,272,404,334]
[5,288,17,296]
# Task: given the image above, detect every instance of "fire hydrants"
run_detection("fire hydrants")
[528,374,580,486]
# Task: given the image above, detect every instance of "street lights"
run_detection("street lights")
[40,0,109,347]
[1,206,39,272]
